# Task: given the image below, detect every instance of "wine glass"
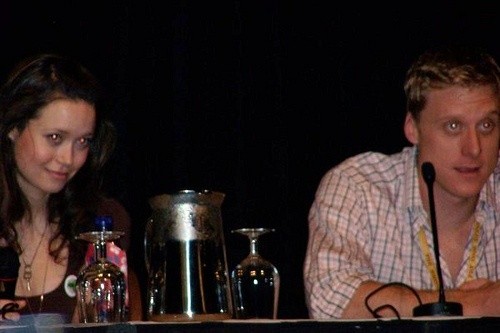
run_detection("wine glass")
[231,228,280,320]
[75,231,128,323]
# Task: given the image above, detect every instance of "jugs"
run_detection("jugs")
[144,191,232,322]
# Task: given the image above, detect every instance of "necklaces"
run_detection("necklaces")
[18,223,48,333]
[10,221,48,292]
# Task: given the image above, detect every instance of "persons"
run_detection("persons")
[0,52,129,323]
[303,44,500,320]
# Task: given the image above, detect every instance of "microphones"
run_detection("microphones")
[413,162,464,317]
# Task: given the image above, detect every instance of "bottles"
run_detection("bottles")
[85,217,128,278]
